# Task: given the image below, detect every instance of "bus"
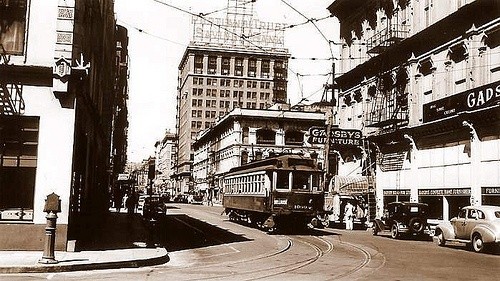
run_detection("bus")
[219,153,333,235]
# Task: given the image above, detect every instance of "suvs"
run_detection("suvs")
[371,200,430,239]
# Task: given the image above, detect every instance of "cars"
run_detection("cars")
[434,205,500,253]
[137,192,205,226]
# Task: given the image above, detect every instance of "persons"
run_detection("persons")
[344,199,356,231]
[108,182,214,220]
